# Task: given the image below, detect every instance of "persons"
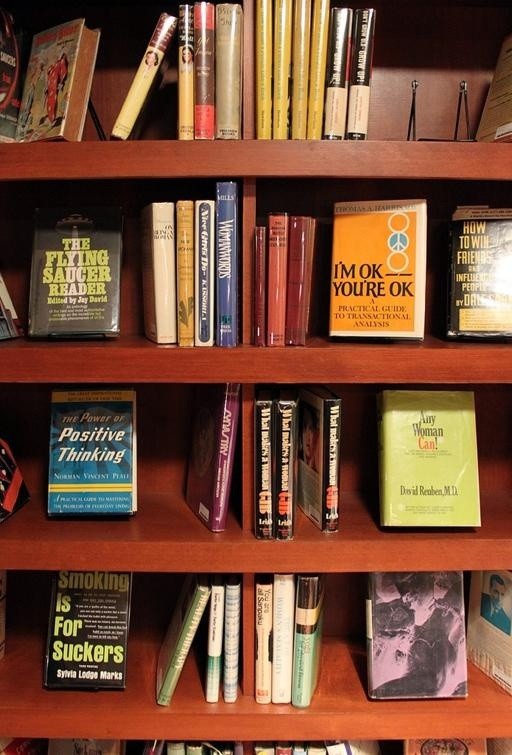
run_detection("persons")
[19,60,44,139]
[47,53,68,127]
[180,44,194,73]
[143,51,158,75]
[298,404,319,471]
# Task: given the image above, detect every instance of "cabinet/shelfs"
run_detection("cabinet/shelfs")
[1,0,512,755]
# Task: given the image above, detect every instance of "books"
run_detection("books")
[1,6,30,143]
[14,18,101,141]
[139,176,329,348]
[1,277,24,339]
[111,12,177,141]
[29,203,124,337]
[467,570,511,694]
[475,28,511,144]
[179,1,375,141]
[330,198,426,342]
[2,568,511,755]
[447,204,511,339]
[0,379,482,550]
